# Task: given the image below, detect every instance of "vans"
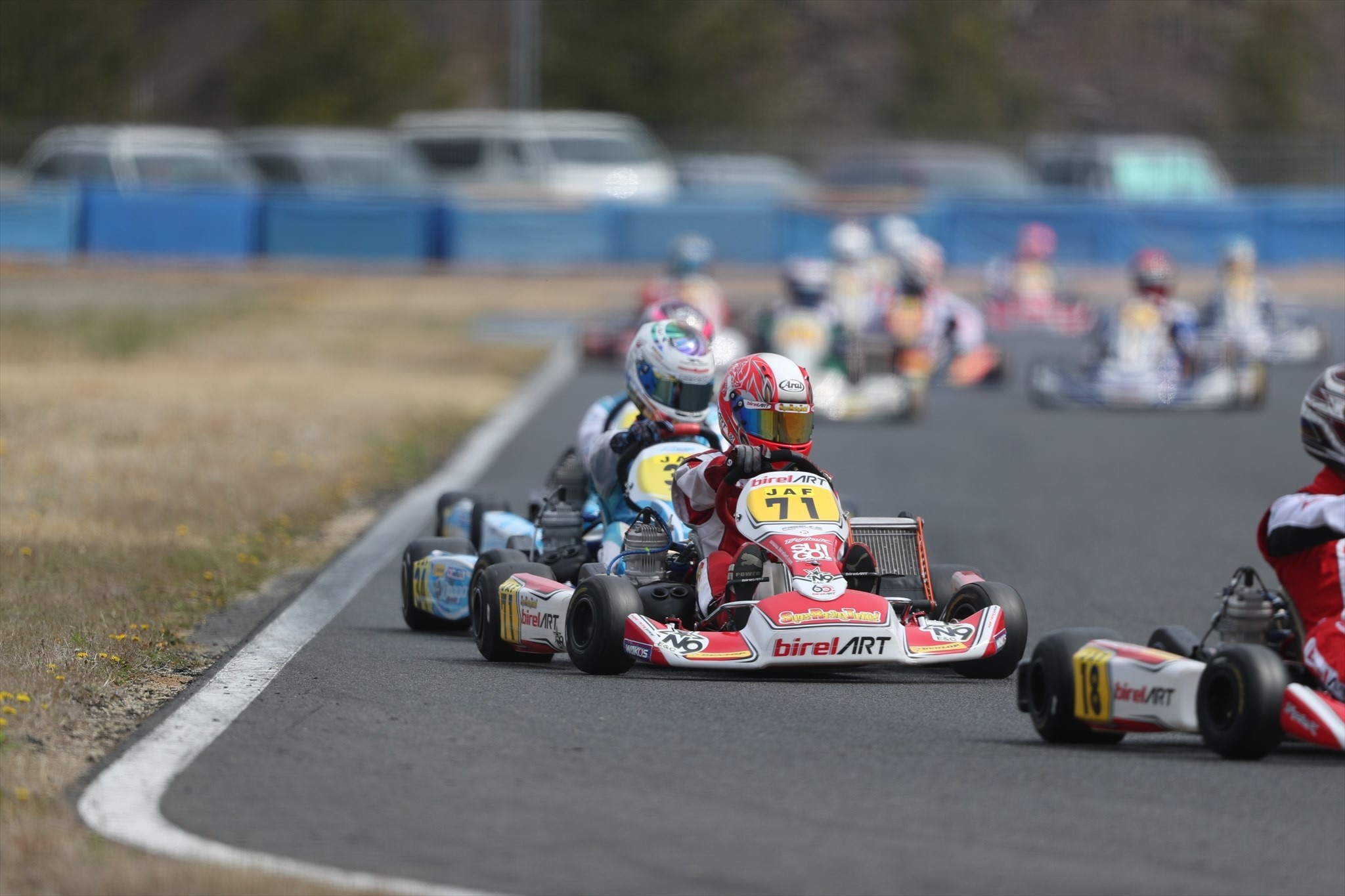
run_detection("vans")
[396,103,679,202]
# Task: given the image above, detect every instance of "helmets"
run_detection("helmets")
[637,298,715,343]
[1301,363,1345,467]
[625,318,714,424]
[1016,222,1058,261]
[665,232,712,273]
[1223,235,1256,275]
[786,256,830,306]
[828,217,875,266]
[896,235,945,296]
[1133,248,1174,298]
[718,352,815,470]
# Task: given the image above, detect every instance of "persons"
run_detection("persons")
[997,224,1270,407]
[672,353,879,632]
[577,300,732,576]
[1259,363,1345,701]
[631,211,985,384]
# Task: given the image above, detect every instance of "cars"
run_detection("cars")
[231,123,442,194]
[811,140,1047,195]
[1040,128,1241,200]
[17,123,271,191]
[683,148,837,204]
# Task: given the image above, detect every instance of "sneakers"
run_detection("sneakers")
[716,541,764,629]
[842,543,878,593]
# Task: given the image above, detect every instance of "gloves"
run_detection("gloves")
[610,418,675,455]
[725,444,771,474]
